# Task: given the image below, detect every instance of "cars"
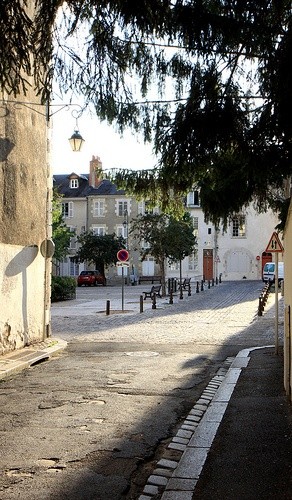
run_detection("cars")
[77,270,107,287]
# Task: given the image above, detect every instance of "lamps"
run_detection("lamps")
[0,98,89,154]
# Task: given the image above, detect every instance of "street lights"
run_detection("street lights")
[123,209,130,285]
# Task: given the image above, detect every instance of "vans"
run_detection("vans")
[263,262,285,287]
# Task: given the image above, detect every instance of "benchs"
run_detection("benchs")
[139,275,163,285]
[142,284,165,300]
[178,278,191,290]
